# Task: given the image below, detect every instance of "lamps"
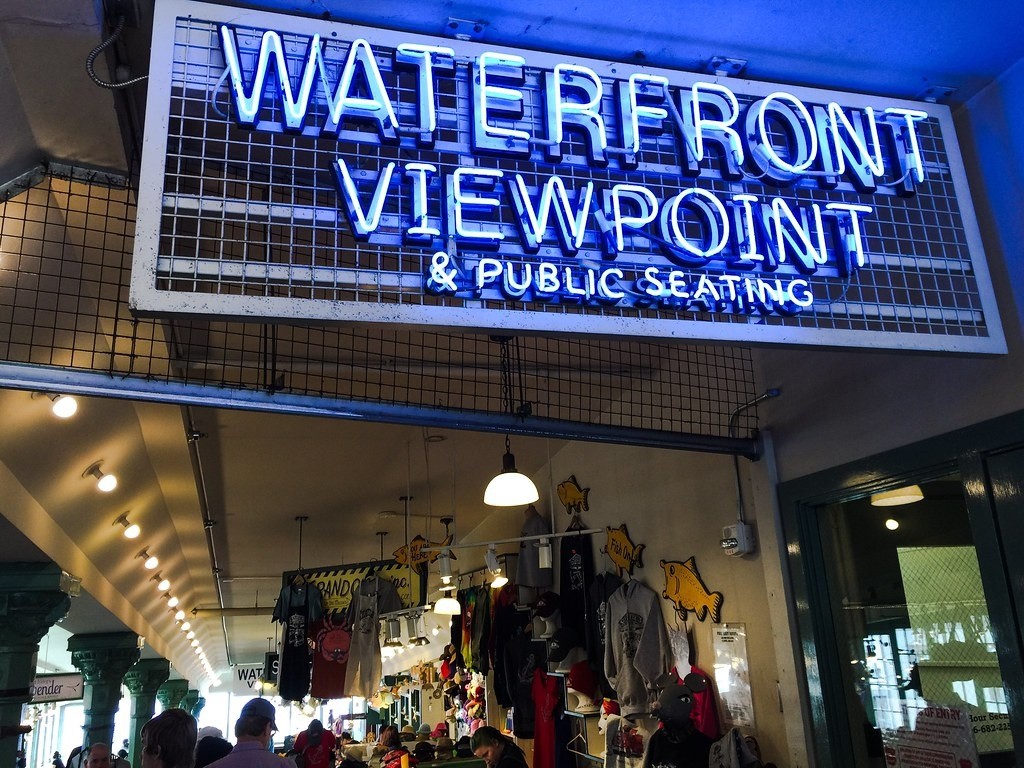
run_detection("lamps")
[484,336,539,507]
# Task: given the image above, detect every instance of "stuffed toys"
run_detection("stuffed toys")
[642,670,712,768]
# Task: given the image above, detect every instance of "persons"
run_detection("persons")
[18,697,534,768]
[898,659,983,768]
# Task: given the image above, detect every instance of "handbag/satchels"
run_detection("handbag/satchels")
[295,755,305,768]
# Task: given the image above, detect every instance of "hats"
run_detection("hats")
[531,592,560,617]
[240,698,279,731]
[412,742,436,752]
[441,644,486,737]
[400,725,416,736]
[380,748,419,768]
[566,659,598,699]
[453,736,471,751]
[417,723,432,733]
[545,628,579,663]
[435,738,454,749]
[436,722,448,732]
